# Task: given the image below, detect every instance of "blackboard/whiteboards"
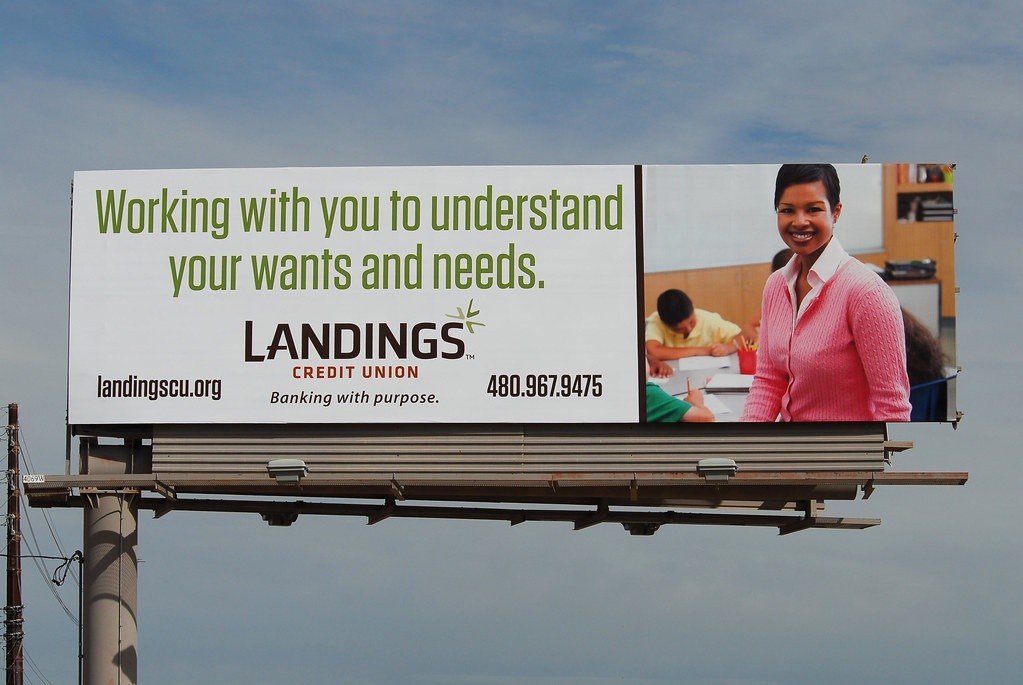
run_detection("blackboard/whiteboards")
[642,163,886,275]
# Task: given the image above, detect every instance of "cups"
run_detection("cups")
[738,347,757,375]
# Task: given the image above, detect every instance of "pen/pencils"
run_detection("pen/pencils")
[687,377,691,396]
[733,334,759,351]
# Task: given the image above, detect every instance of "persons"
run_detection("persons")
[645,246,949,422]
[739,164,914,421]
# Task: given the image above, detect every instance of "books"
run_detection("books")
[898,164,954,222]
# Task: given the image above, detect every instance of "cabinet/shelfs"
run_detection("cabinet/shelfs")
[880,164,954,317]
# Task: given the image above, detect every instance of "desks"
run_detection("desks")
[648,355,781,421]
[879,277,941,340]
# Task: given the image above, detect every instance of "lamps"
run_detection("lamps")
[695,457,739,484]
[267,457,310,484]
